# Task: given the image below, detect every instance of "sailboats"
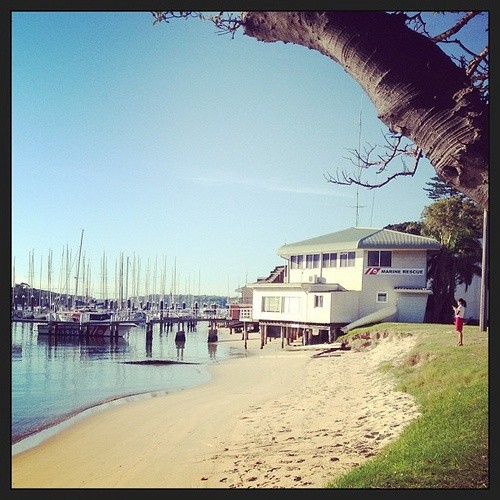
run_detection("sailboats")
[13,228,226,336]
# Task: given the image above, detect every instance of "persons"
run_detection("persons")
[452,297,467,346]
